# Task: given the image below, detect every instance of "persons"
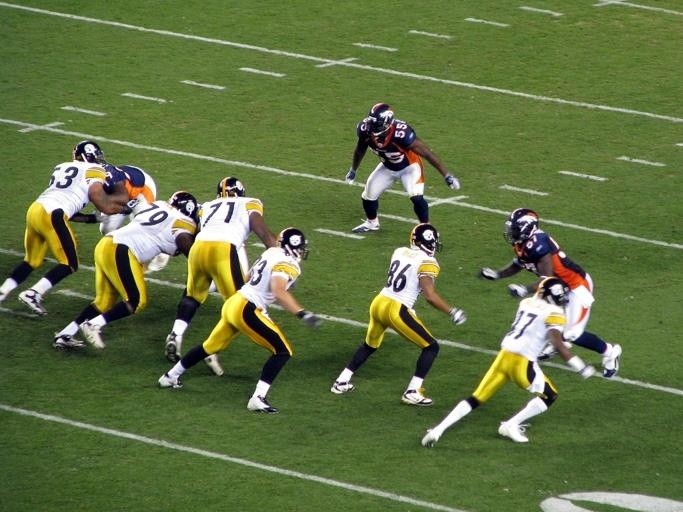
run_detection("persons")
[0,142,220,354]
[479,209,623,378]
[344,101,463,234]
[330,223,466,408]
[418,274,596,447]
[156,228,322,413]
[165,176,276,377]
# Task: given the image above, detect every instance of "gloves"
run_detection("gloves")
[481,268,500,281]
[449,308,466,324]
[579,365,594,379]
[345,167,356,184]
[445,174,460,190]
[506,285,528,298]
[295,310,322,327]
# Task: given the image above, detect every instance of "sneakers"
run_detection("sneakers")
[539,340,573,360]
[0,288,278,414]
[330,380,528,446]
[602,344,622,378]
[351,221,381,232]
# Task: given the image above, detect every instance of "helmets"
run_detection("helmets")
[74,140,309,262]
[366,103,395,133]
[537,277,573,308]
[502,210,539,246]
[410,223,443,257]
